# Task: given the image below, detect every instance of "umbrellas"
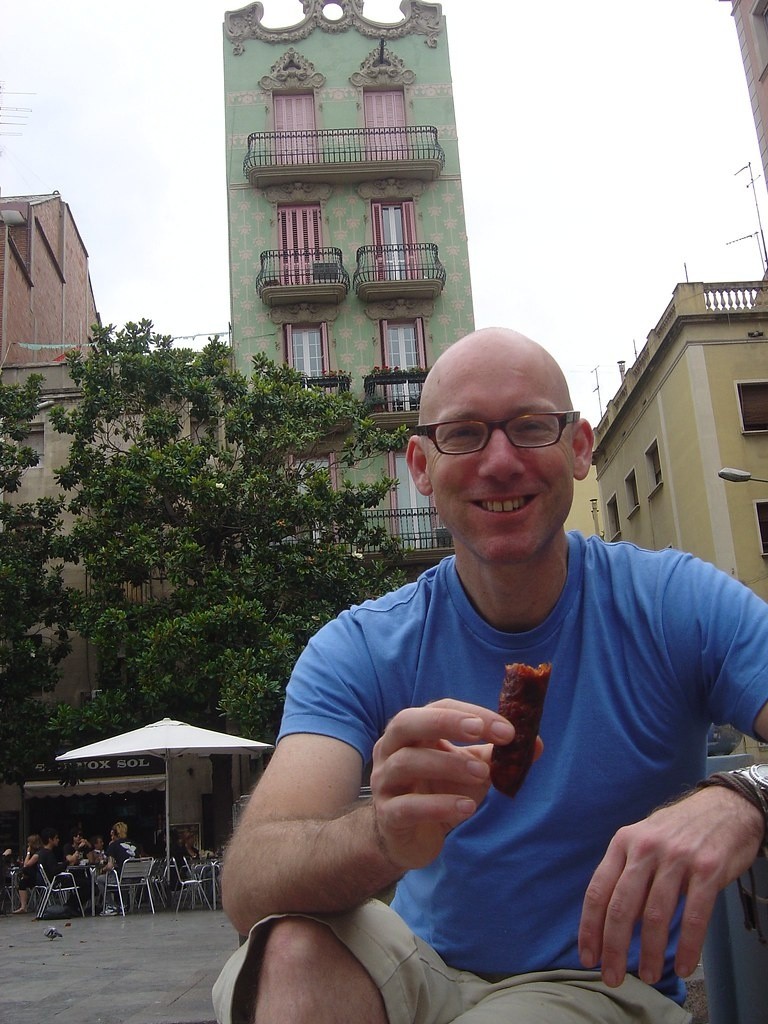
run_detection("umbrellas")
[55,718,275,908]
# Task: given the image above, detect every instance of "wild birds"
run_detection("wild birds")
[43,926,63,941]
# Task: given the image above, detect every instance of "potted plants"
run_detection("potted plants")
[368,394,386,412]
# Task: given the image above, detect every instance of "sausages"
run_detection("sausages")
[489,663,552,799]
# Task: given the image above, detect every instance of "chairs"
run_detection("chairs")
[0,854,222,922]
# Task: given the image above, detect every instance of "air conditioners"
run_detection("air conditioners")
[310,260,343,284]
[433,526,454,547]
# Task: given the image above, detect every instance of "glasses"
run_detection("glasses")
[414,410,581,455]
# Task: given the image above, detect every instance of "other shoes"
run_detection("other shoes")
[13,908,28,913]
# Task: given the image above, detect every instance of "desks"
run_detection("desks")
[189,858,219,908]
[67,864,105,916]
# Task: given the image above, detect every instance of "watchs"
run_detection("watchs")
[727,763,768,803]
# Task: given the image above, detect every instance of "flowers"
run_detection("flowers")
[321,370,355,383]
[370,365,433,377]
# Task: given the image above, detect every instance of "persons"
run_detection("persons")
[211,326,768,1024]
[0,822,233,917]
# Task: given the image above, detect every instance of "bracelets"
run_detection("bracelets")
[652,771,768,820]
[27,851,30,855]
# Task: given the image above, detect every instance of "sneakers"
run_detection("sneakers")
[119,905,130,915]
[99,904,118,916]
